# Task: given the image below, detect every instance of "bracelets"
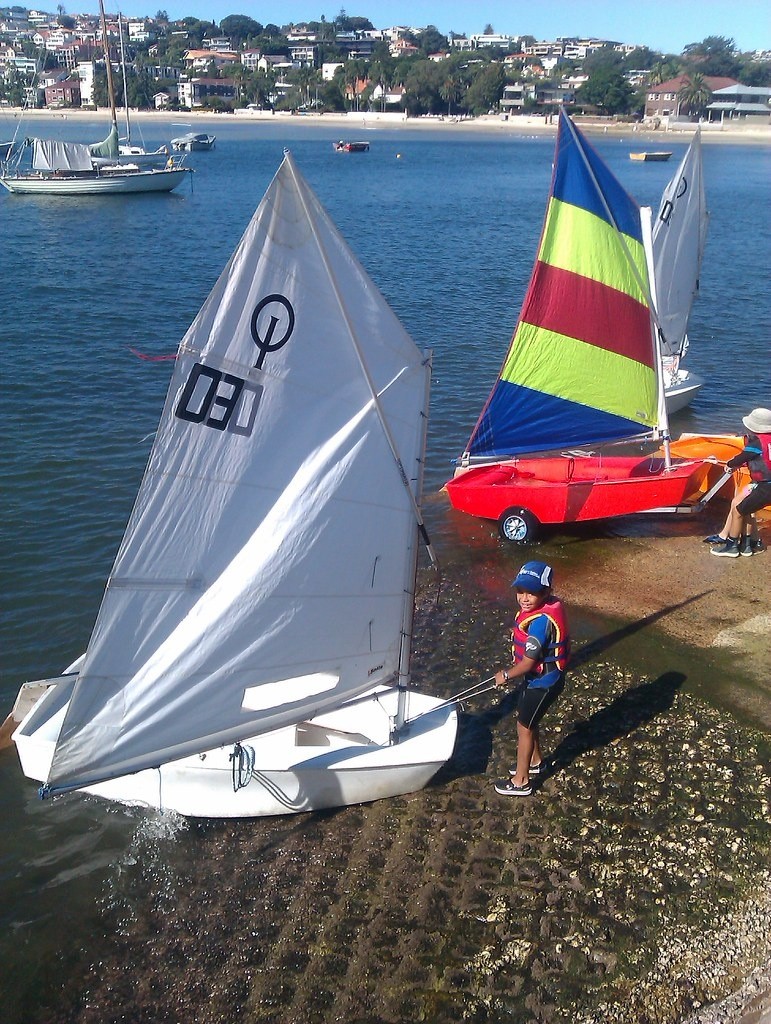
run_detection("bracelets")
[503,670,509,682]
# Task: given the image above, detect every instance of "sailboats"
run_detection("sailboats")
[650,125,707,417]
[0,0,197,196]
[9,145,458,819]
[441,105,716,524]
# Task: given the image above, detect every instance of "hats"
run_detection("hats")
[742,408,771,434]
[511,561,553,591]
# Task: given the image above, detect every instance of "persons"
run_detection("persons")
[495,562,571,795]
[705,408,771,557]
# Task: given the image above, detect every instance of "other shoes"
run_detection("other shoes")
[736,544,752,556]
[703,535,726,544]
[710,546,739,558]
[751,539,764,555]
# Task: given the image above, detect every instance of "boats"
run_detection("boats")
[333,140,370,153]
[170,123,217,153]
[630,152,673,161]
[658,435,770,519]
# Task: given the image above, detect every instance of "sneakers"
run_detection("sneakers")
[494,777,532,795]
[508,760,547,775]
